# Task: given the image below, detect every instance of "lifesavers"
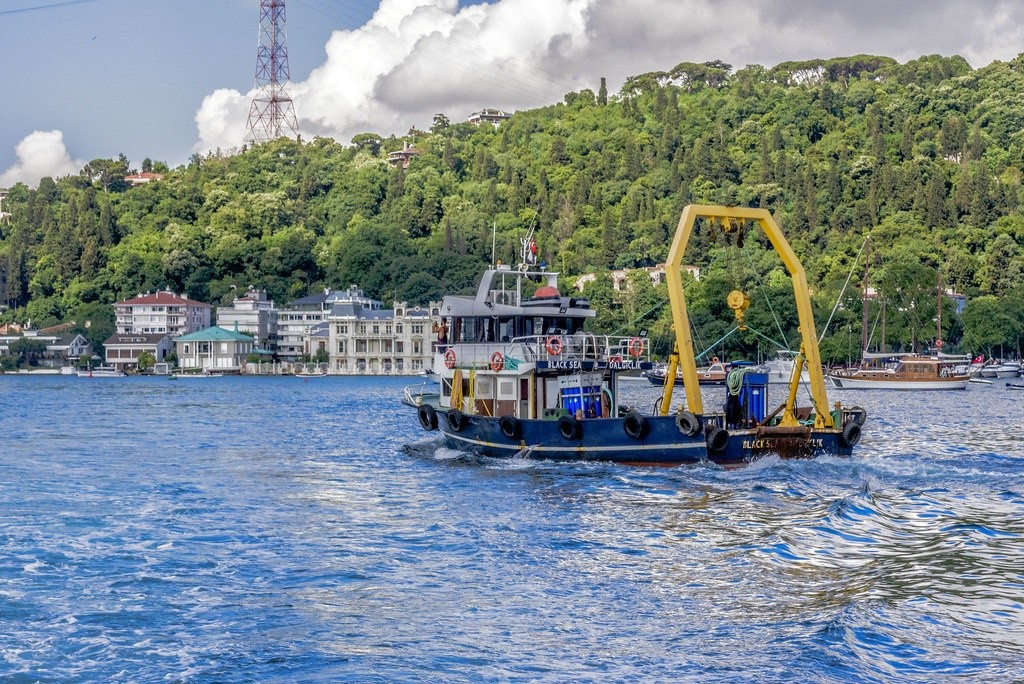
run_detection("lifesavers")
[935,340,942,347]
[849,405,867,427]
[545,336,563,355]
[675,411,700,437]
[623,411,646,439]
[713,357,719,363]
[556,414,579,441]
[707,427,730,452]
[490,351,503,371]
[444,350,456,368]
[842,420,861,448]
[417,404,437,432]
[499,415,520,439]
[629,338,644,357]
[447,408,465,432]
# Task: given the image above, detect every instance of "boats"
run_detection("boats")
[171,370,224,378]
[419,201,634,387]
[167,372,179,380]
[967,364,983,379]
[643,357,732,386]
[746,351,812,384]
[59,366,78,376]
[76,369,125,377]
[995,362,1024,380]
[951,365,971,376]
[401,203,867,469]
[981,365,1001,378]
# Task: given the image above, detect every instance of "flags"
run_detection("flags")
[972,353,984,364]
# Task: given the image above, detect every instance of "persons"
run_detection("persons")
[602,390,609,418]
[432,318,448,354]
[941,365,950,377]
[486,329,494,341]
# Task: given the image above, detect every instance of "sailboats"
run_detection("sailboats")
[825,236,993,389]
